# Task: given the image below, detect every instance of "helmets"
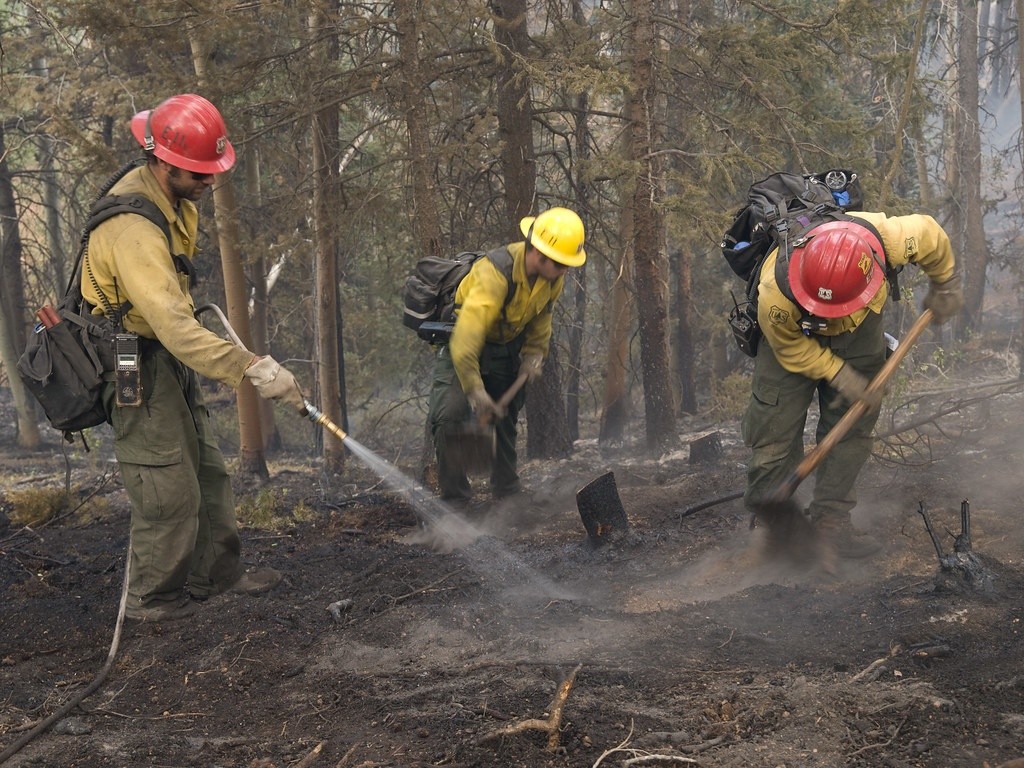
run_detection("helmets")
[520,206,589,267]
[129,93,238,174]
[788,218,885,319]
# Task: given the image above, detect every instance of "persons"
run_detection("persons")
[428,207,587,520]
[82,95,304,618]
[740,212,961,576]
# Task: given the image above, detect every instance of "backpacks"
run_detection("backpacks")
[715,168,902,337]
[397,244,518,348]
[16,190,199,453]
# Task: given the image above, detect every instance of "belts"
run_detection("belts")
[96,339,153,374]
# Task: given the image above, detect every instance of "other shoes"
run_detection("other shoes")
[501,488,554,515]
[760,532,833,573]
[123,599,200,626]
[810,504,883,558]
[221,568,283,598]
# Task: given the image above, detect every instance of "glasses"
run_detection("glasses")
[187,170,211,182]
[549,259,570,270]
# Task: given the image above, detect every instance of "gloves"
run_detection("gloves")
[922,276,967,328]
[827,363,887,419]
[520,351,548,383]
[469,386,506,432]
[242,352,311,418]
